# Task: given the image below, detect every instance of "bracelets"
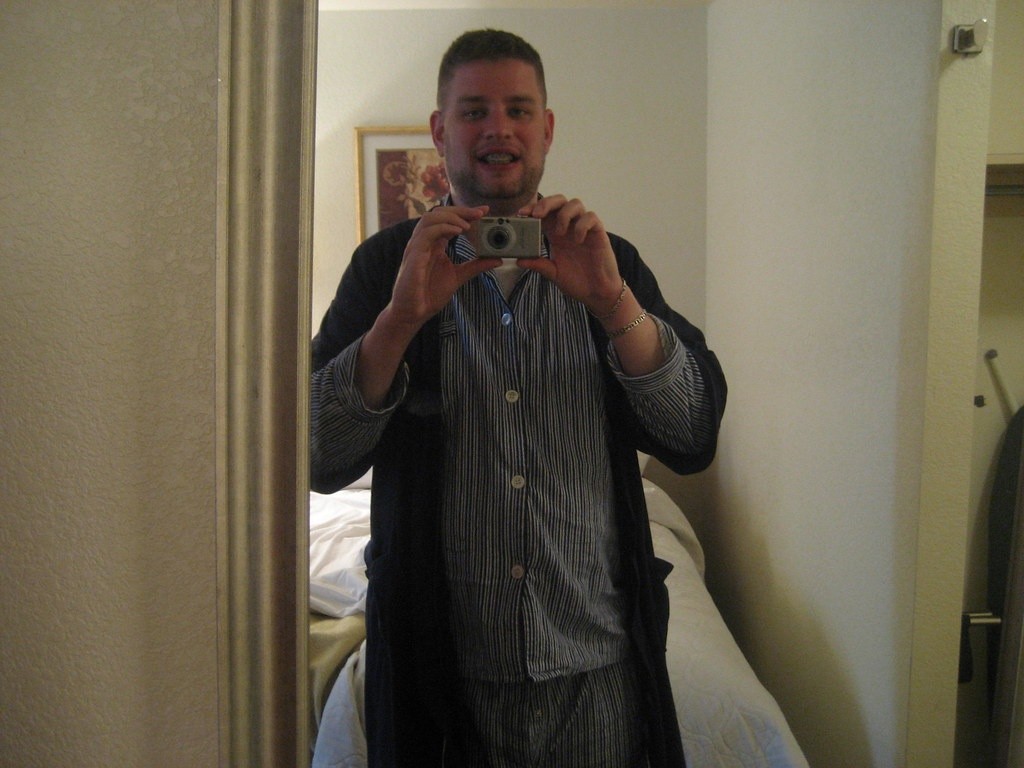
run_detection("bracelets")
[606,308,646,339]
[585,278,627,319]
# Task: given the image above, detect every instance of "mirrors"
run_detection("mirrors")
[218,0,1024,768]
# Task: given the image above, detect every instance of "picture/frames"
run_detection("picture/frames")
[354,125,451,245]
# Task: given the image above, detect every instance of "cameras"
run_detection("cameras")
[478,215,544,259]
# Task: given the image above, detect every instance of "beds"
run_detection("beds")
[310,450,810,768]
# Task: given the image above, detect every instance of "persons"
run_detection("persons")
[309,28,727,768]
